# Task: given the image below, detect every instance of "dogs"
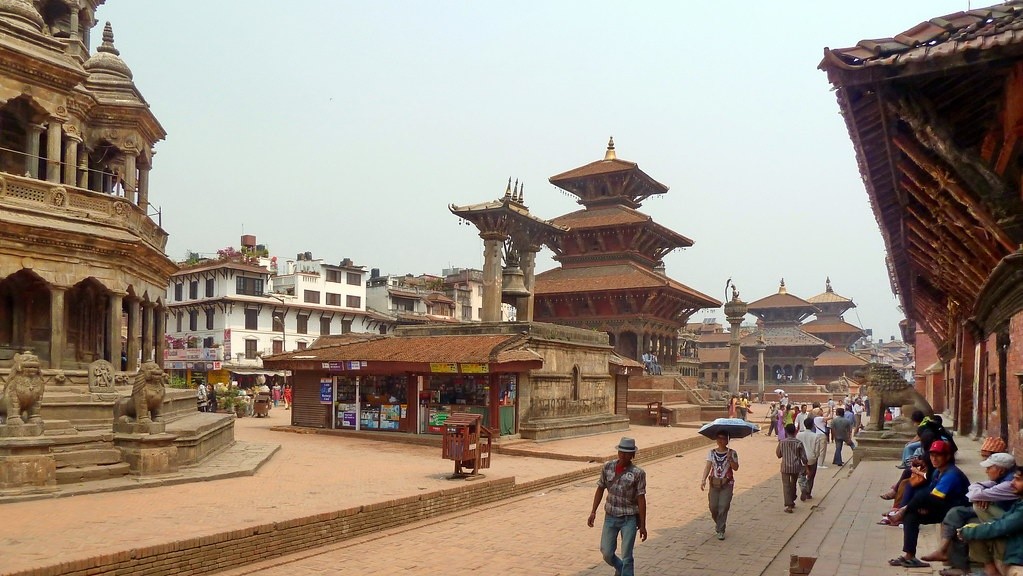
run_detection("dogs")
[892,455,928,510]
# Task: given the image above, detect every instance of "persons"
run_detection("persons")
[727,389,753,420]
[206,383,218,413]
[829,408,856,467]
[810,407,833,470]
[795,417,820,502]
[775,424,807,514]
[270,382,292,410]
[194,378,209,412]
[765,390,893,443]
[876,411,1022,576]
[642,351,663,377]
[775,369,794,385]
[699,430,739,540]
[587,436,648,576]
[120,350,128,362]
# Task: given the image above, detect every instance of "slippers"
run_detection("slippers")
[876,518,899,526]
[880,492,896,499]
[901,556,930,567]
[889,556,908,565]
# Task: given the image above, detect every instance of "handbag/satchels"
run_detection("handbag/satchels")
[824,427,831,435]
[709,475,728,489]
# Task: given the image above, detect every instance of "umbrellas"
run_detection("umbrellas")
[773,389,784,395]
[697,418,759,462]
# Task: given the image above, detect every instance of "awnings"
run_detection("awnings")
[224,367,291,376]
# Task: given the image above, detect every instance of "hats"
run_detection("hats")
[981,436,1007,454]
[615,437,639,453]
[927,440,950,454]
[980,453,1016,470]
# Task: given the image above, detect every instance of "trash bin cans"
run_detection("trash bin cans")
[253,392,271,418]
[647,401,672,425]
[442,412,491,481]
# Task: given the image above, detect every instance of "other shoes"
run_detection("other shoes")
[783,507,793,513]
[716,529,724,541]
[806,494,813,499]
[800,488,808,501]
[896,464,906,469]
[882,510,899,516]
